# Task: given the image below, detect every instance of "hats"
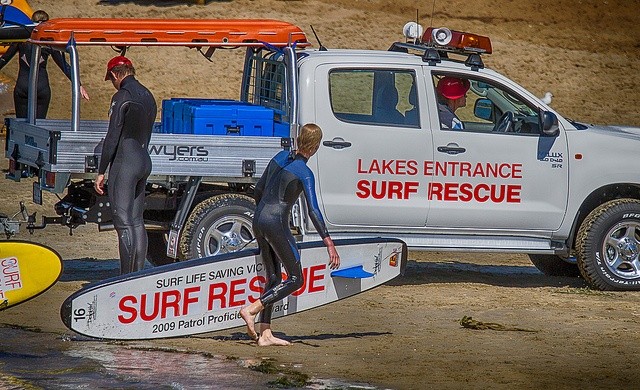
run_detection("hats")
[104,56,133,81]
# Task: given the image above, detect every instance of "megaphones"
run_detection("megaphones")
[403,22,423,38]
[432,27,452,46]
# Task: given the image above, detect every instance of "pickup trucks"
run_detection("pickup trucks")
[5,20,640,290]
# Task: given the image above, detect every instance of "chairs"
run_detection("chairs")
[405,85,420,125]
[375,85,405,124]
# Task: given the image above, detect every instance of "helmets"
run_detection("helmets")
[437,76,471,101]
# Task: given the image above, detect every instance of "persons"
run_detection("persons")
[437,76,470,129]
[94,56,158,277]
[0,9,91,179]
[240,124,341,345]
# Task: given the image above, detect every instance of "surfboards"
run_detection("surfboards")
[0,239,64,311]
[61,237,408,340]
